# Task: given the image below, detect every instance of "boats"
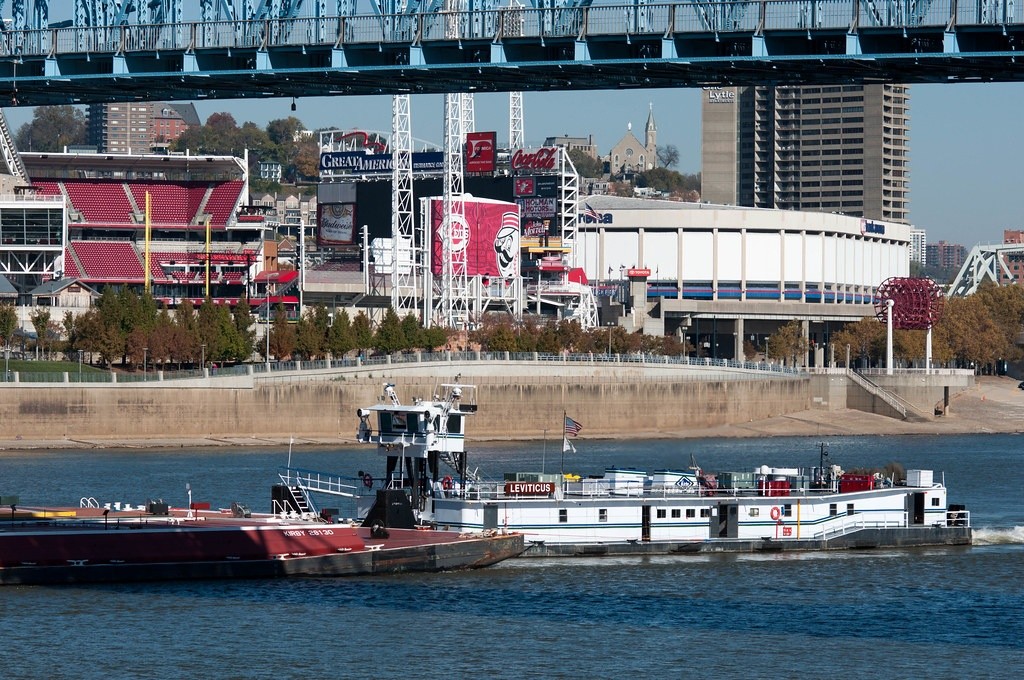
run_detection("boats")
[271,373,974,559]
[0,490,524,585]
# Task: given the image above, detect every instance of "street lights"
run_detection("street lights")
[681,326,688,365]
[607,322,615,359]
[5,349,11,382]
[78,350,83,382]
[733,332,738,368]
[764,337,770,370]
[142,347,149,382]
[201,345,206,371]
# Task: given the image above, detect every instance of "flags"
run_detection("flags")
[608,264,658,274]
[585,202,602,220]
[565,417,583,437]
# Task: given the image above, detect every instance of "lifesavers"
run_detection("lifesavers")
[770,507,780,519]
[363,472,373,487]
[442,475,453,490]
